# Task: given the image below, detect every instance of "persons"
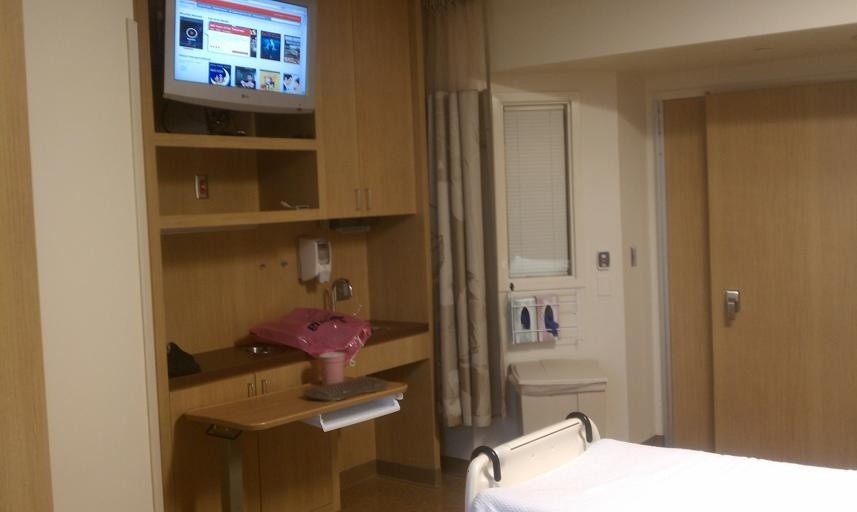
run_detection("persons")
[214,30,300,93]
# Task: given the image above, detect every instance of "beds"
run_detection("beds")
[464,414,853,512]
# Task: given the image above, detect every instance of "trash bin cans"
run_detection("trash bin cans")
[507,359,608,437]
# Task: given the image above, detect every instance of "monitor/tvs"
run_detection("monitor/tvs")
[154,0,316,115]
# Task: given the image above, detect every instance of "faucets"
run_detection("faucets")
[329,275,353,312]
